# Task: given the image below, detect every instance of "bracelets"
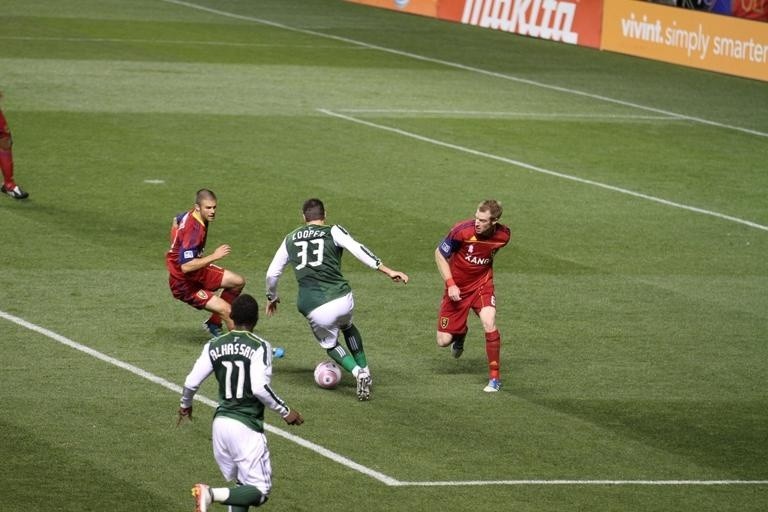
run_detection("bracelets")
[446,278,456,287]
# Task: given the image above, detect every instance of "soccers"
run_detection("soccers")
[314,360,341,389]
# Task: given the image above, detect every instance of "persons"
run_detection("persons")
[178,293,304,512]
[0,91,29,199]
[434,200,511,392]
[265,198,409,402]
[164,189,244,336]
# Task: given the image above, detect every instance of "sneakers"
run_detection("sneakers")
[0,183,28,200]
[203,318,226,337]
[484,380,499,394]
[191,484,212,512]
[451,329,467,359]
[355,369,372,401]
